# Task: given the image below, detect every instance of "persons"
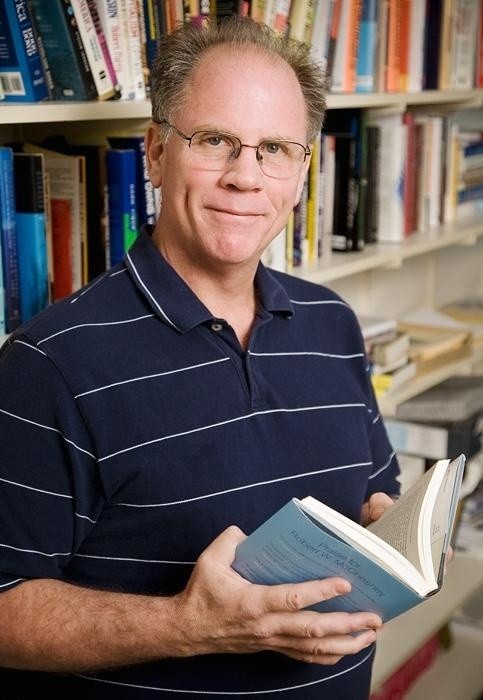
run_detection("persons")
[0,16,401,700]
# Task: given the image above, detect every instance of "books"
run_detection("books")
[354,301,483,397]
[380,374,483,482]
[231,453,466,637]
[259,112,483,275]
[0,0,483,105]
[0,128,162,335]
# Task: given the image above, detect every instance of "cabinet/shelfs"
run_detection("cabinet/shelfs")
[0,0,483,699]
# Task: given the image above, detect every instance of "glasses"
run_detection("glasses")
[163,118,312,181]
